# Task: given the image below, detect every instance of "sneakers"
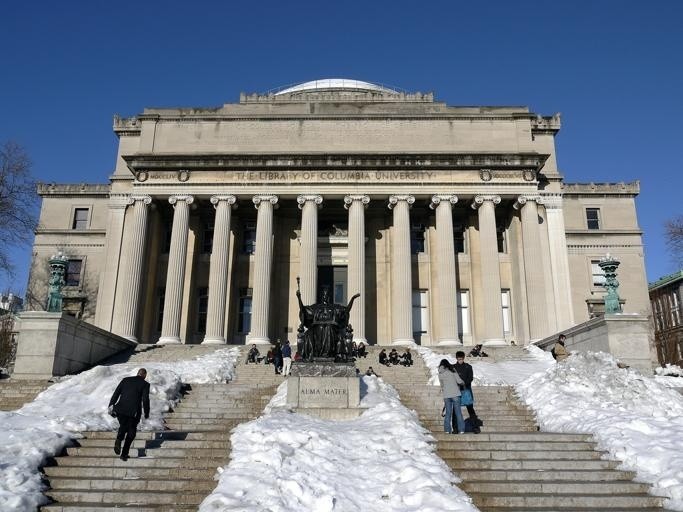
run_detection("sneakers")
[112,437,131,459]
[444,426,481,434]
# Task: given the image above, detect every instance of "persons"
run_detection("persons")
[438,344,483,434]
[263,339,291,377]
[245,344,260,364]
[554,335,569,365]
[366,367,378,378]
[389,349,400,365]
[358,342,366,358]
[399,349,411,367]
[351,342,357,358]
[379,349,390,367]
[109,369,150,459]
[356,369,361,377]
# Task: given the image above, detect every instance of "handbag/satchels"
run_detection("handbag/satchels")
[458,382,475,407]
